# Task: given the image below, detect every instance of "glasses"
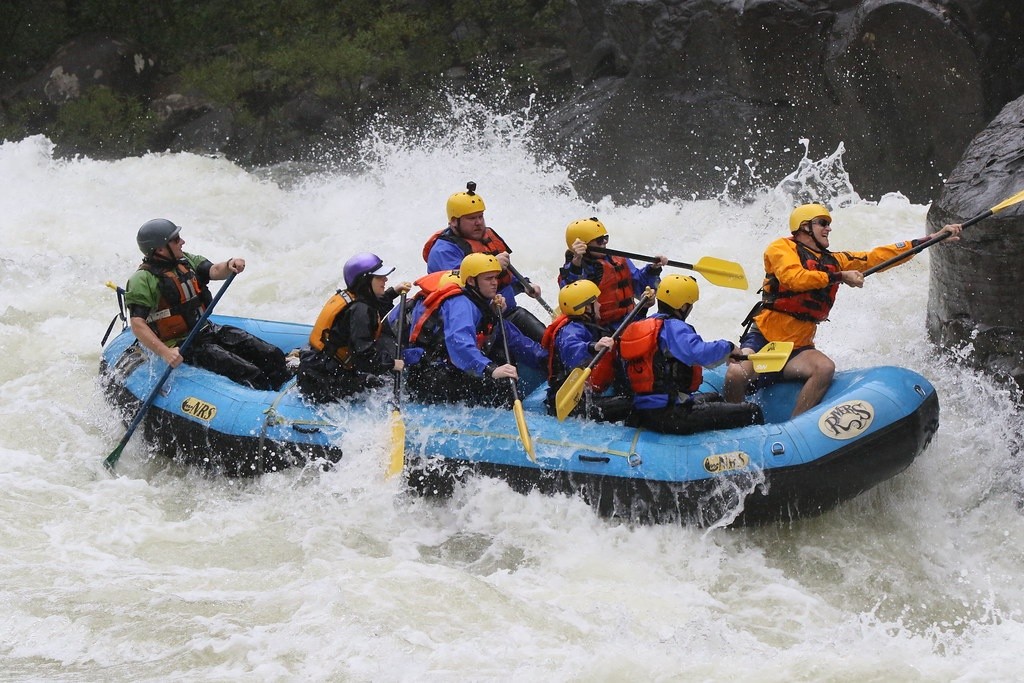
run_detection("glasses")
[168,236,180,245]
[596,235,609,245]
[799,218,830,227]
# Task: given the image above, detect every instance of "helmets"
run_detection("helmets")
[789,203,832,235]
[136,218,181,256]
[460,251,502,285]
[656,274,700,309]
[558,279,602,316]
[566,218,607,252]
[343,252,382,288]
[448,190,486,222]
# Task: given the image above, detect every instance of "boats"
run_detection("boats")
[98,312,940,528]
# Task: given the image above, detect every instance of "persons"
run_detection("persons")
[423,191,546,347]
[557,219,668,332]
[545,281,656,419]
[409,253,546,407]
[299,252,411,399]
[725,204,961,415]
[622,275,763,435]
[125,219,289,389]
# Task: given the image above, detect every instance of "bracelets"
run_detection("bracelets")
[227,258,232,267]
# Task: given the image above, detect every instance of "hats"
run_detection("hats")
[369,262,397,276]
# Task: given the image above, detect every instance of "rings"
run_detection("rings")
[858,276,861,279]
[243,265,245,266]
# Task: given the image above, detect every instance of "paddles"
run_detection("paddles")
[554,281,660,420]
[851,189,1024,280]
[102,263,243,473]
[378,287,406,474]
[501,253,554,320]
[492,291,539,462]
[575,241,755,291]
[721,338,795,375]
